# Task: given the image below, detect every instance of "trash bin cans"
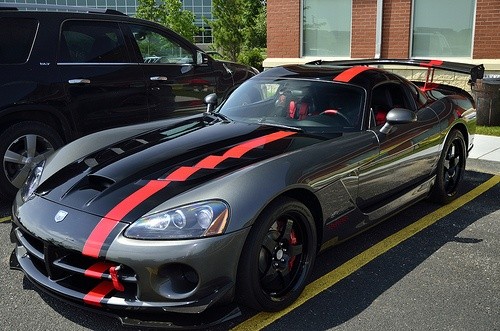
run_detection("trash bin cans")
[467,73,500,126]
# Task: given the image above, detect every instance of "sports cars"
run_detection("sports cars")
[9,58,485,330]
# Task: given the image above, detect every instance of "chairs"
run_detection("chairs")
[277,82,318,122]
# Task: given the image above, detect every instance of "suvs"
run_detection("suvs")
[0,2,267,201]
